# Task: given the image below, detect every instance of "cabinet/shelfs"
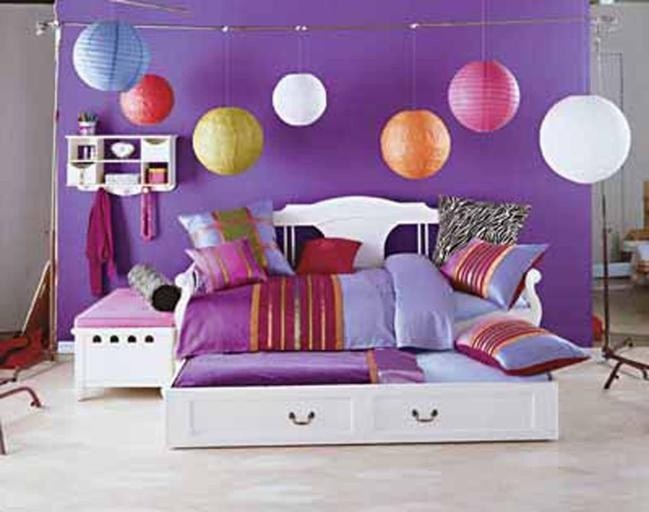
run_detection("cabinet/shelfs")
[64,132,180,198]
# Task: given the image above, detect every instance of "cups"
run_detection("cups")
[79,121,95,135]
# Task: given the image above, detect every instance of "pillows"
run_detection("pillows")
[432,194,592,377]
[176,198,364,293]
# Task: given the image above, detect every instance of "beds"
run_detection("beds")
[157,194,559,447]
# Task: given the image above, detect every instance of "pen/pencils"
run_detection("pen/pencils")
[77,111,98,121]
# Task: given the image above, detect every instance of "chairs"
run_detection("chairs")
[0,260,51,455]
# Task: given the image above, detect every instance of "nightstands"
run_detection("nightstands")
[70,287,177,401]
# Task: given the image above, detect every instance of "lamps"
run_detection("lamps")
[271,40,330,128]
[190,60,265,179]
[70,1,154,95]
[379,40,452,184]
[536,12,633,184]
[118,61,176,127]
[448,5,521,132]
